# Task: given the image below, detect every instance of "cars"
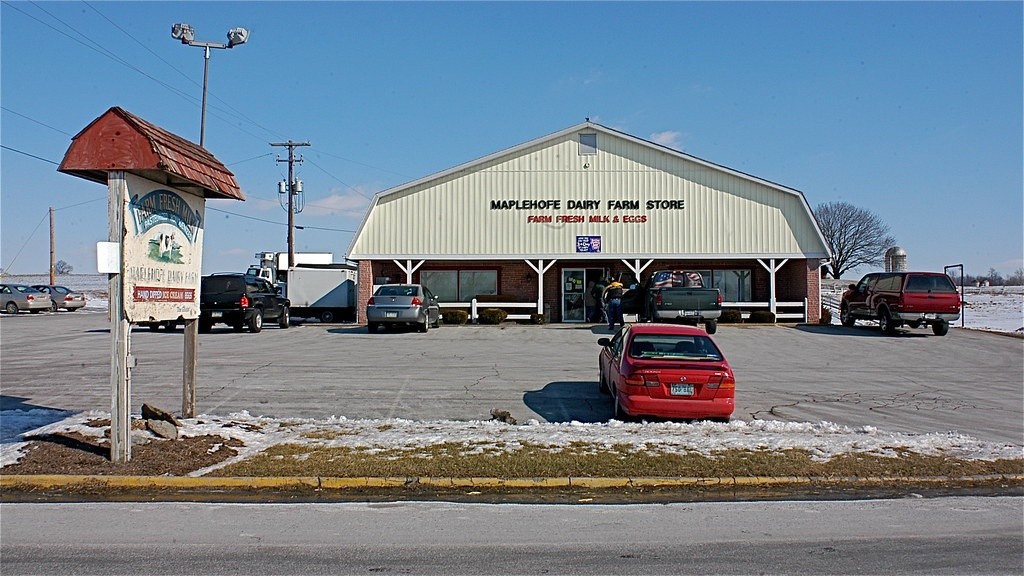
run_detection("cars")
[21,285,86,312]
[597,323,735,423]
[0,283,52,315]
[367,283,441,333]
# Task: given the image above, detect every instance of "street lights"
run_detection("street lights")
[170,22,252,151]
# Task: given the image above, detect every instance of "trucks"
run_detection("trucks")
[245,252,359,323]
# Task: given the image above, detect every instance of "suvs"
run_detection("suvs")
[840,271,960,336]
[199,272,290,333]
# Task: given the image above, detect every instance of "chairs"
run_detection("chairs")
[675,341,696,353]
[632,341,655,355]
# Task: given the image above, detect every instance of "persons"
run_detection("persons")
[586,276,625,330]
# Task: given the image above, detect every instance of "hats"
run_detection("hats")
[608,276,615,282]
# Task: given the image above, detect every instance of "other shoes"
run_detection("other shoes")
[587,317,590,322]
[608,327,613,330]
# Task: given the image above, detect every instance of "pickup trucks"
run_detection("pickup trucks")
[630,270,723,335]
